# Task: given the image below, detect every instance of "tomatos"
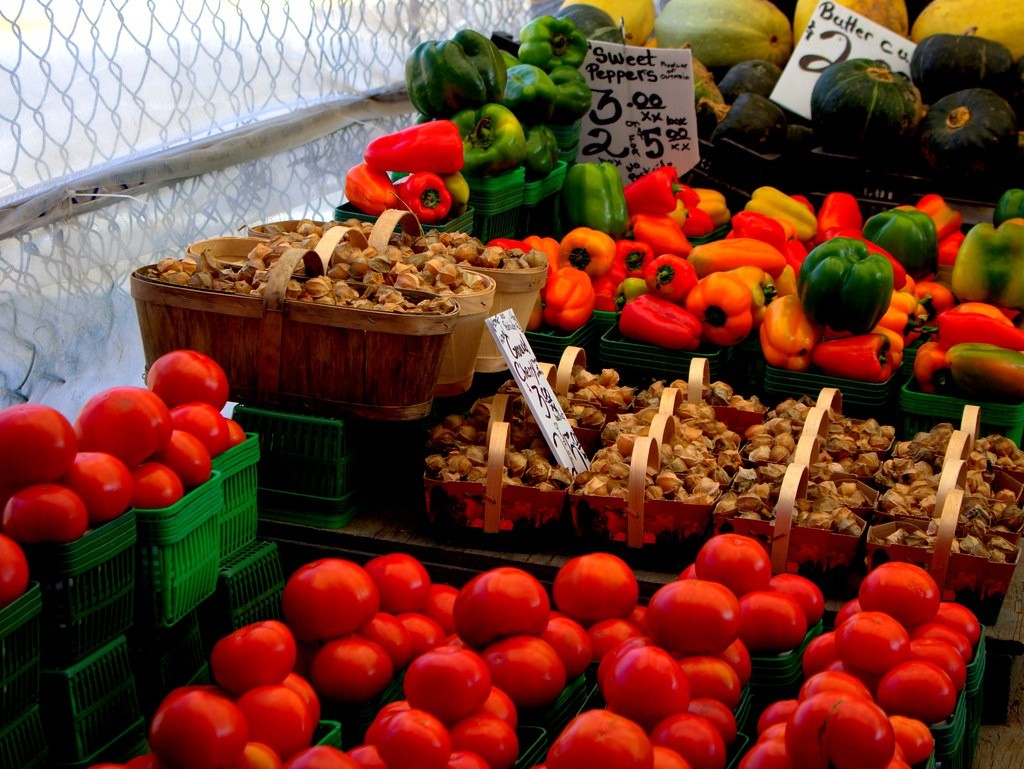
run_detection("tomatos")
[0,350,982,769]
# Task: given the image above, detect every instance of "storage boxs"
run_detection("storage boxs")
[0,117,1024,769]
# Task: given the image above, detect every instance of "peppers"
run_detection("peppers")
[345,15,1024,401]
